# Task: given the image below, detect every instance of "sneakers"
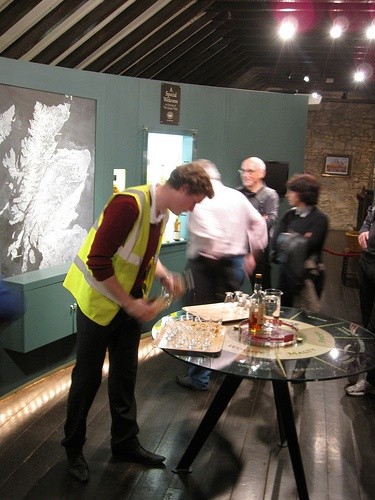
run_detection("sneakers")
[346,379,375,396]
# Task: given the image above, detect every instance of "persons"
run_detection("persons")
[266,174,329,394]
[346,196,375,396]
[173,159,268,391]
[231,157,279,295]
[61,162,215,483]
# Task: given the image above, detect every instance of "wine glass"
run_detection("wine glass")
[165,311,223,350]
[223,290,259,317]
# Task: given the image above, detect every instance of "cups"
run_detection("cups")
[262,288,284,334]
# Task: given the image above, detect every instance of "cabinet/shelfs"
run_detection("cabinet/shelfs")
[0,282,77,354]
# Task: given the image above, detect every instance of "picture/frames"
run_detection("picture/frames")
[321,153,352,178]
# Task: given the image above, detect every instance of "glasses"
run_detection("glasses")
[237,169,258,175]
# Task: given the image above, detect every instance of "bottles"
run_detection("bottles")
[249,274,265,335]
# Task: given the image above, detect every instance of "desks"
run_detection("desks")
[151,303,375,500]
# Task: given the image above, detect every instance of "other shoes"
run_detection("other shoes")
[291,371,305,379]
[68,454,89,483]
[112,442,166,464]
[176,374,208,391]
